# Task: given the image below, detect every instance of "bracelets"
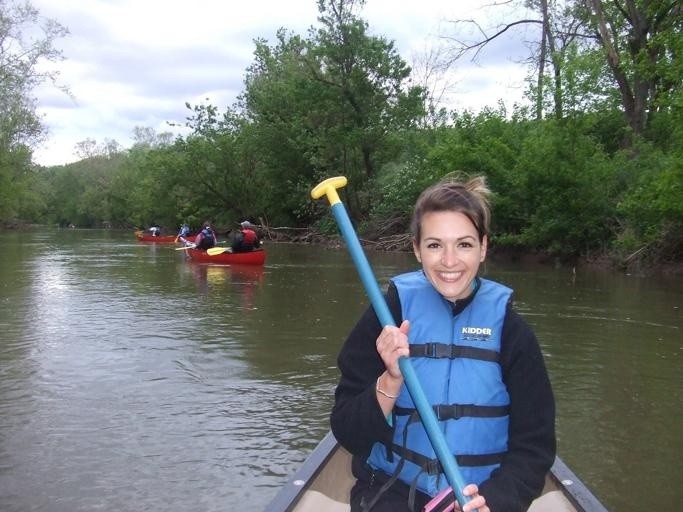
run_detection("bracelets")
[376,372,400,400]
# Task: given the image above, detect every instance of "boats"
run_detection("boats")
[261,427,609,512]
[134,231,197,242]
[180,237,265,266]
[182,259,262,286]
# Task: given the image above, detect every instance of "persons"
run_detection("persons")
[328,168,557,512]
[192,220,217,252]
[229,220,265,253]
[147,223,190,237]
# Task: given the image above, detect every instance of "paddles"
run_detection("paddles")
[175,240,226,251]
[206,240,264,256]
